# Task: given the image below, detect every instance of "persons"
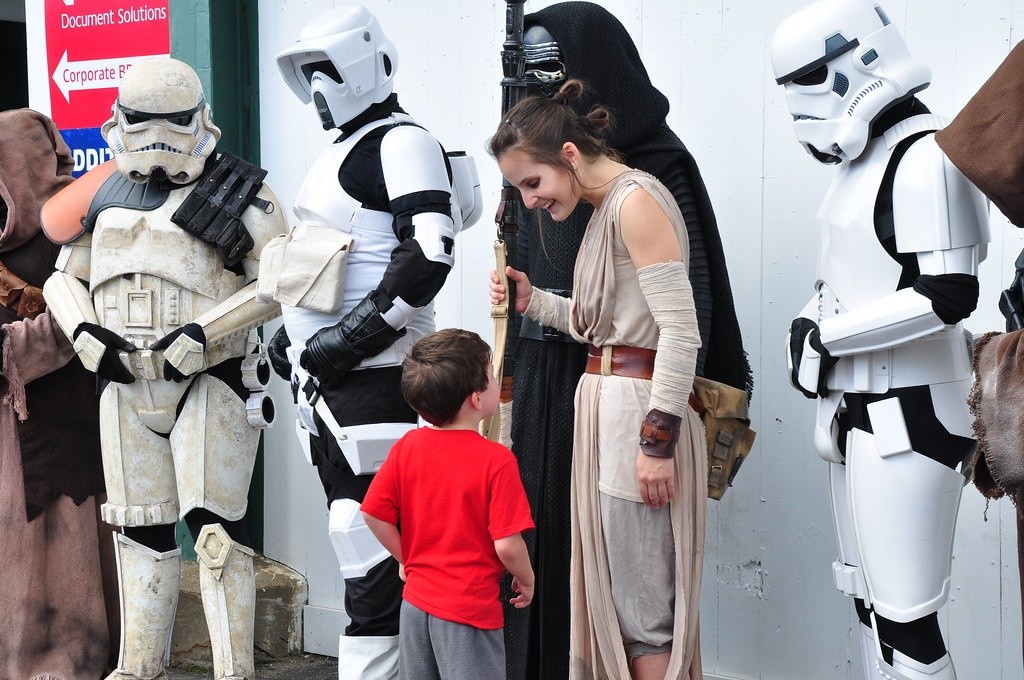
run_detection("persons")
[490,79,707,680]
[934,37,1024,665]
[500,0,756,680]
[1,109,120,680]
[41,54,291,680]
[772,0,992,680]
[359,328,535,680]
[265,4,482,680]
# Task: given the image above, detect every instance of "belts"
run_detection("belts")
[584,344,658,382]
